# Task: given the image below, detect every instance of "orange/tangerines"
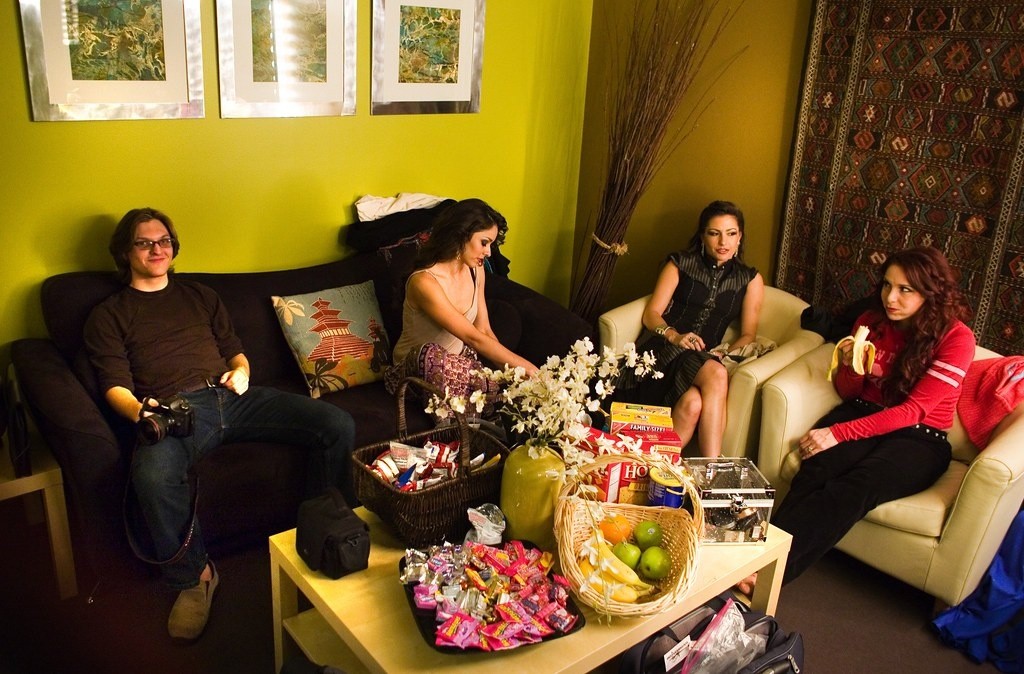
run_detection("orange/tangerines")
[599,515,631,546]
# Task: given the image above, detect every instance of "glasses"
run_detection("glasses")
[128,237,177,251]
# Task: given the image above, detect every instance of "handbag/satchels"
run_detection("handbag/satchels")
[637,603,806,674]
[296,486,369,580]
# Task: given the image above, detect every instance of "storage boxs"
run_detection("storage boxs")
[610,401,671,422]
[561,423,624,504]
[610,411,673,437]
[617,431,682,507]
[682,455,776,547]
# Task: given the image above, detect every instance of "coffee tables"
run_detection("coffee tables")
[268,504,793,674]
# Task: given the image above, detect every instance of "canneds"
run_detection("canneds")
[647,467,685,510]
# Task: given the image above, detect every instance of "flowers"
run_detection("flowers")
[425,336,664,518]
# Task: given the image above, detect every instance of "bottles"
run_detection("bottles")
[7,401,32,477]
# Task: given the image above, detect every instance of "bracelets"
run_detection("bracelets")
[654,324,677,337]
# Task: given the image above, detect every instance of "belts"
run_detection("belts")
[177,374,221,394]
[855,398,948,441]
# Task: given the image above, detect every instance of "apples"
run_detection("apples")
[613,543,640,570]
[640,546,672,579]
[633,522,661,549]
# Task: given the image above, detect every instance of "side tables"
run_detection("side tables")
[0,408,79,600]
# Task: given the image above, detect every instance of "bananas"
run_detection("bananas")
[580,530,661,602]
[828,326,875,385]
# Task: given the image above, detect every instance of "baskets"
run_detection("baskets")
[348,377,516,550]
[553,455,706,619]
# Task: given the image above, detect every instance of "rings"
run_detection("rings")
[690,337,696,343]
[807,447,812,452]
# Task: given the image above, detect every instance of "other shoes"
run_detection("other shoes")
[168,559,219,640]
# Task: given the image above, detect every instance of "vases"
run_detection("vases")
[499,440,567,551]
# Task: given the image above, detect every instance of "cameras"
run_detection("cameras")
[137,394,196,447]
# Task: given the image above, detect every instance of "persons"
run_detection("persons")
[730,245,975,599]
[604,199,763,459]
[80,207,354,640]
[384,198,550,428]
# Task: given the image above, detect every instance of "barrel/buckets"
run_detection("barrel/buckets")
[647,467,686,508]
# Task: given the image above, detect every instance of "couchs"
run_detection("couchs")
[11,242,593,581]
[596,278,824,464]
[757,343,1024,619]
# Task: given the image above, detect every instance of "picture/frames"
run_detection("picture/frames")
[216,0,358,119]
[369,0,486,116]
[18,0,206,122]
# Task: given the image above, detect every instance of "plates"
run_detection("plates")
[399,540,586,654]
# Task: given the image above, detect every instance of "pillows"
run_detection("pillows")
[270,280,393,399]
